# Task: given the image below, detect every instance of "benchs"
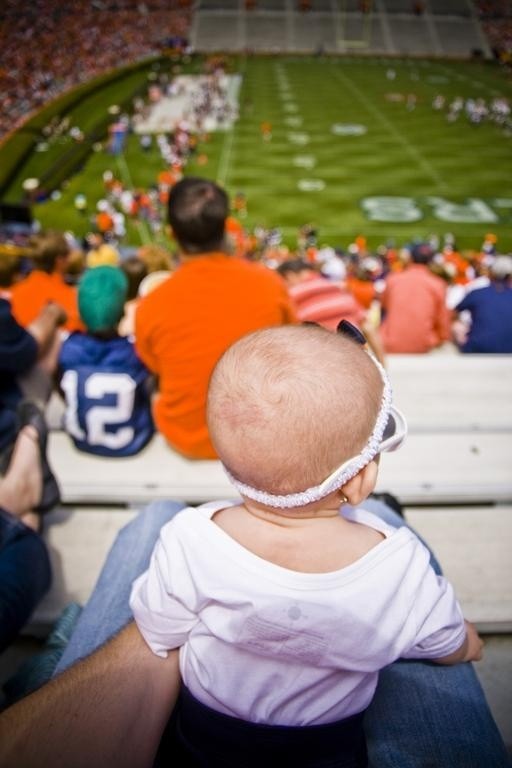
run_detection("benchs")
[30,434,511,636]
[384,351,510,434]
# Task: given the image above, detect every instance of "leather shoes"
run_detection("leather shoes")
[18,401,59,530]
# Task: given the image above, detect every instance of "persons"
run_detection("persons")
[128,325,485,768]
[0,406,60,658]
[106,52,231,167]
[2,1,194,139]
[117,177,298,465]
[0,493,508,768]
[434,95,511,128]
[1,163,512,352]
[56,266,155,459]
[474,1,511,71]
[0,292,69,469]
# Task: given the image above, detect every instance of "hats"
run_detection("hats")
[412,242,432,262]
[490,255,512,280]
[77,265,131,333]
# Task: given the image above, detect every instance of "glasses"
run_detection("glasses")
[318,403,406,498]
